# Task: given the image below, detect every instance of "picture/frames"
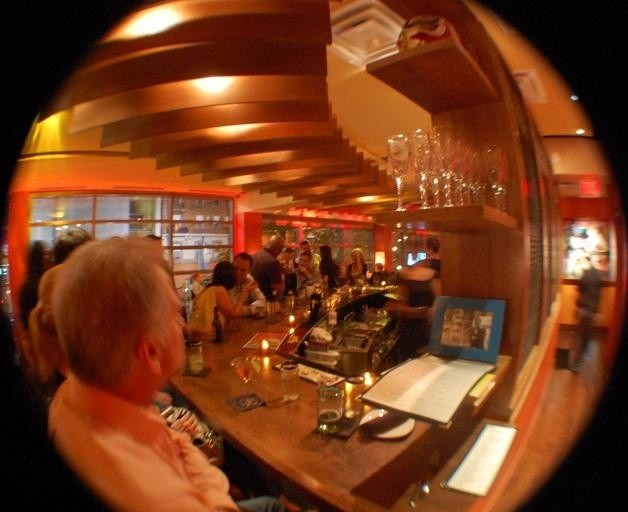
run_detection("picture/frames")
[428,295,507,364]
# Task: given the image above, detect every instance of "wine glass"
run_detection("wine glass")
[387,133,410,211]
[415,127,518,210]
[230,356,259,407]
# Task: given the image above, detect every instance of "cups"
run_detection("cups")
[279,361,301,400]
[344,375,364,420]
[266,300,276,323]
[317,384,344,435]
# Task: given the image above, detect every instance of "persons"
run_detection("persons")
[469,315,487,348]
[18,229,438,510]
[569,257,601,374]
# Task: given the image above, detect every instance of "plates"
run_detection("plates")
[358,408,414,439]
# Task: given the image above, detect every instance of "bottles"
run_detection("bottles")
[187,339,203,375]
[285,290,294,314]
